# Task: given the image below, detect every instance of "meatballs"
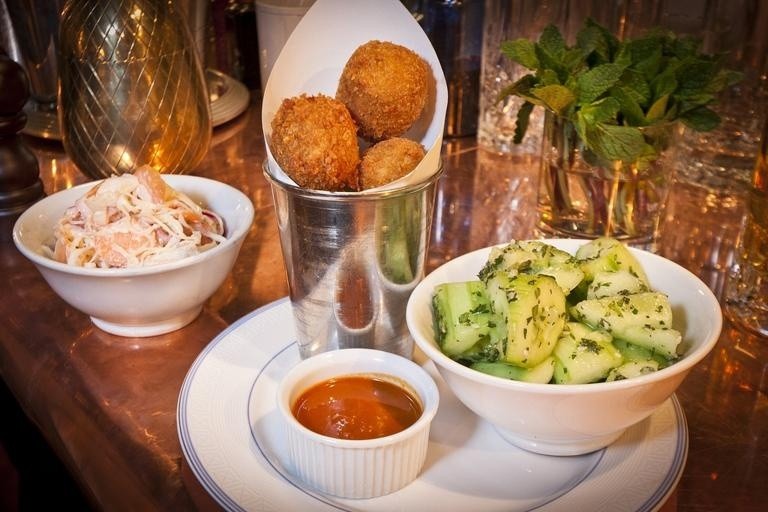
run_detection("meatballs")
[268,39,432,190]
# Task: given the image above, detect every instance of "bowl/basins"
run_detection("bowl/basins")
[12,174,256,338]
[280,346,441,497]
[407,238,722,458]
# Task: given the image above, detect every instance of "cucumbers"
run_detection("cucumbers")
[428,237,682,383]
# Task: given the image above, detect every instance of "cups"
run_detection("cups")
[633,95,767,342]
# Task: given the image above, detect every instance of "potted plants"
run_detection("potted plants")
[495,20,745,241]
